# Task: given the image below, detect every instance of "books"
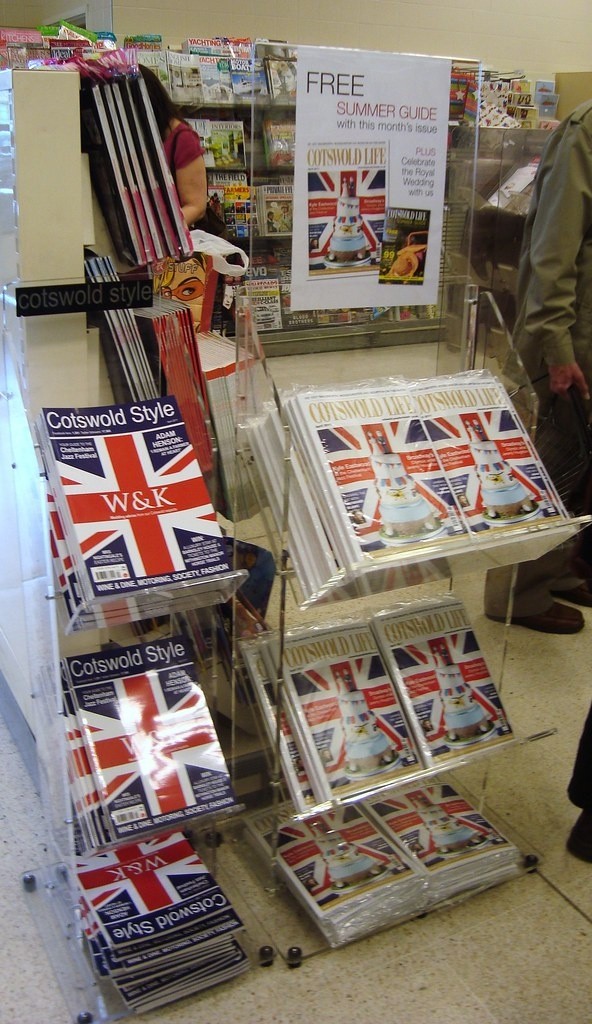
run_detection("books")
[247,378,570,602]
[240,599,514,815]
[33,374,251,1014]
[126,36,560,335]
[83,75,270,522]
[241,775,519,950]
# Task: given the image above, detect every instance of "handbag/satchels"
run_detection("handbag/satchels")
[187,229,249,278]
[531,388,592,509]
[170,129,227,242]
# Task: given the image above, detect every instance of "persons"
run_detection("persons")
[138,63,208,226]
[567,706,592,864]
[483,101,592,632]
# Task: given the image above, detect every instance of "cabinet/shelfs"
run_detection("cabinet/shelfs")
[4,274,280,1024]
[231,39,591,970]
[177,101,555,358]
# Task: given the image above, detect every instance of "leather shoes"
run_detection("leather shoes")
[552,580,592,607]
[487,600,584,634]
